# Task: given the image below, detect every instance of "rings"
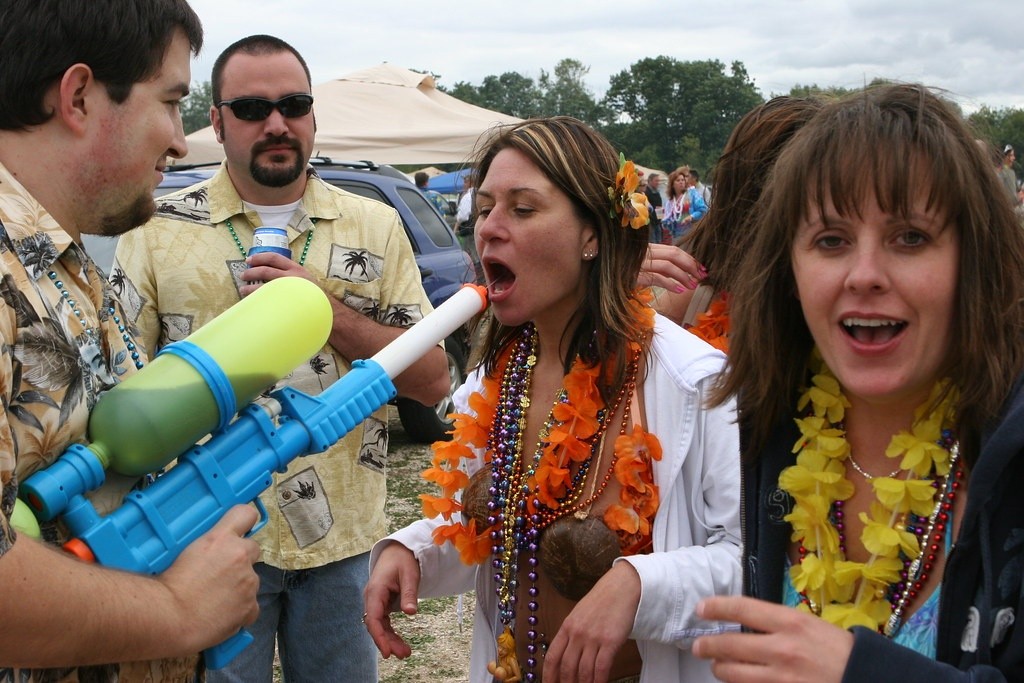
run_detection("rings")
[360,613,368,625]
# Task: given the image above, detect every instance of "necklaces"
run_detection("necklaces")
[225,218,317,267]
[49,271,165,483]
[688,282,734,353]
[418,278,654,682]
[670,192,687,222]
[778,365,967,638]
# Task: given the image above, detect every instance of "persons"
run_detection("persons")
[453,174,487,287]
[692,78,1024,683]
[640,181,653,214]
[0,0,262,683]
[660,171,708,253]
[976,139,1024,221]
[107,34,455,683]
[360,112,740,683]
[676,166,711,207]
[644,172,663,245]
[635,96,822,354]
[414,172,452,221]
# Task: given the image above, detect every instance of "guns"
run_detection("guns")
[3,274,489,672]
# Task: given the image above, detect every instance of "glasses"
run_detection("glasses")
[216,94,313,120]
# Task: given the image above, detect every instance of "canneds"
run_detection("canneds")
[252,226,291,250]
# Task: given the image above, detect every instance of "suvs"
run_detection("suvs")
[77,156,476,445]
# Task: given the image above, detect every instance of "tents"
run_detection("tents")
[160,60,530,165]
[408,166,478,196]
[631,161,668,193]
